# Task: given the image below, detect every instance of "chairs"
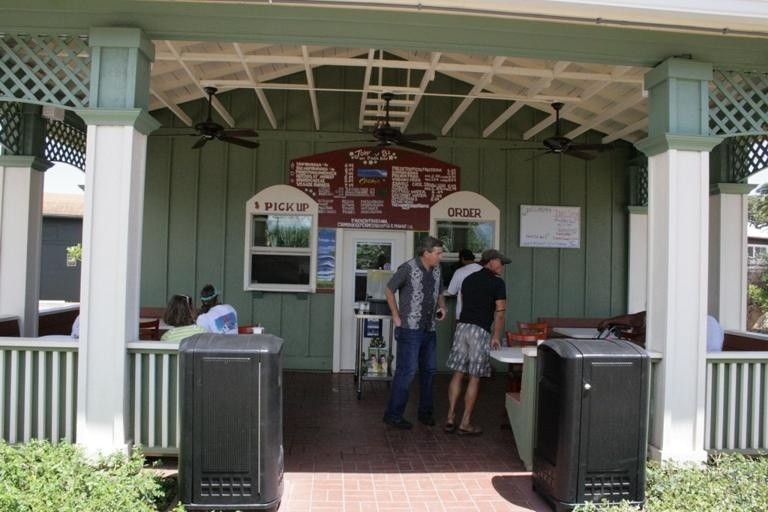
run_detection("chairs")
[489,310,726,477]
[137,319,162,341]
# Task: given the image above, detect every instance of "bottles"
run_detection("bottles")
[70,314,79,343]
[380,354,385,363]
[370,354,376,368]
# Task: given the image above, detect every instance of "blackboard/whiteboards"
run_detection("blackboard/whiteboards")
[520,205,582,249]
[353,272,395,317]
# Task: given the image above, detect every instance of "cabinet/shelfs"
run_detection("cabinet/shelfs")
[350,307,402,399]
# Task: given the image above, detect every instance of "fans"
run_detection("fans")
[146,85,262,152]
[323,89,440,156]
[501,101,609,164]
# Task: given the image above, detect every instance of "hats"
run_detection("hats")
[482,249,512,265]
[200,288,223,301]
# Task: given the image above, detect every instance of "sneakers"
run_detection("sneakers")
[419,416,435,426]
[383,415,412,429]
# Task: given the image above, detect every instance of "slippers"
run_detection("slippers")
[457,423,484,434]
[444,417,460,431]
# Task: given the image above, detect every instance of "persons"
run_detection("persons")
[161,294,207,343]
[443,249,512,435]
[383,236,449,430]
[378,354,388,372]
[196,284,239,335]
[368,354,377,373]
[444,249,484,320]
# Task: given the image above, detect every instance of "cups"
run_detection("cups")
[252,327,264,334]
[353,308,359,314]
